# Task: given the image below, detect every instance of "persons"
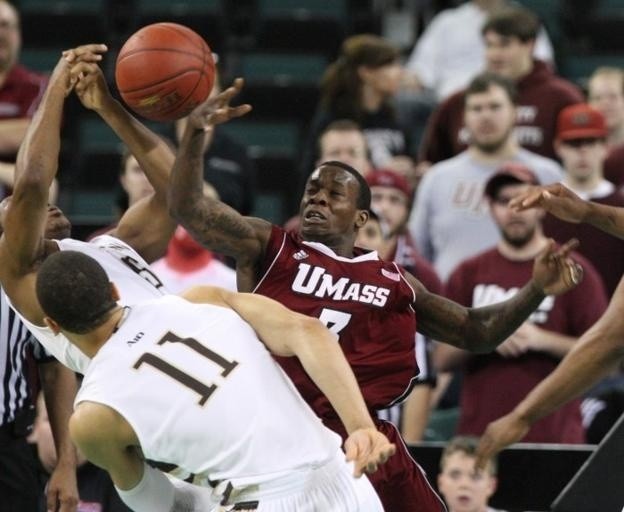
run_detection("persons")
[114,64,257,268]
[406,72,566,286]
[0,292,79,512]
[0,43,213,512]
[62,46,584,512]
[35,251,397,511]
[414,11,584,182]
[315,121,374,183]
[93,141,236,300]
[437,436,505,511]
[353,208,437,445]
[542,106,623,303]
[288,38,418,216]
[586,67,623,184]
[406,0,555,103]
[472,183,623,459]
[282,170,443,297]
[1,1,51,158]
[400,1,555,163]
[433,163,606,444]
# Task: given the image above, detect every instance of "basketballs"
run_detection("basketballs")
[114,22,215,121]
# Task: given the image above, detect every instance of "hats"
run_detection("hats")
[557,104,608,140]
[483,167,538,193]
